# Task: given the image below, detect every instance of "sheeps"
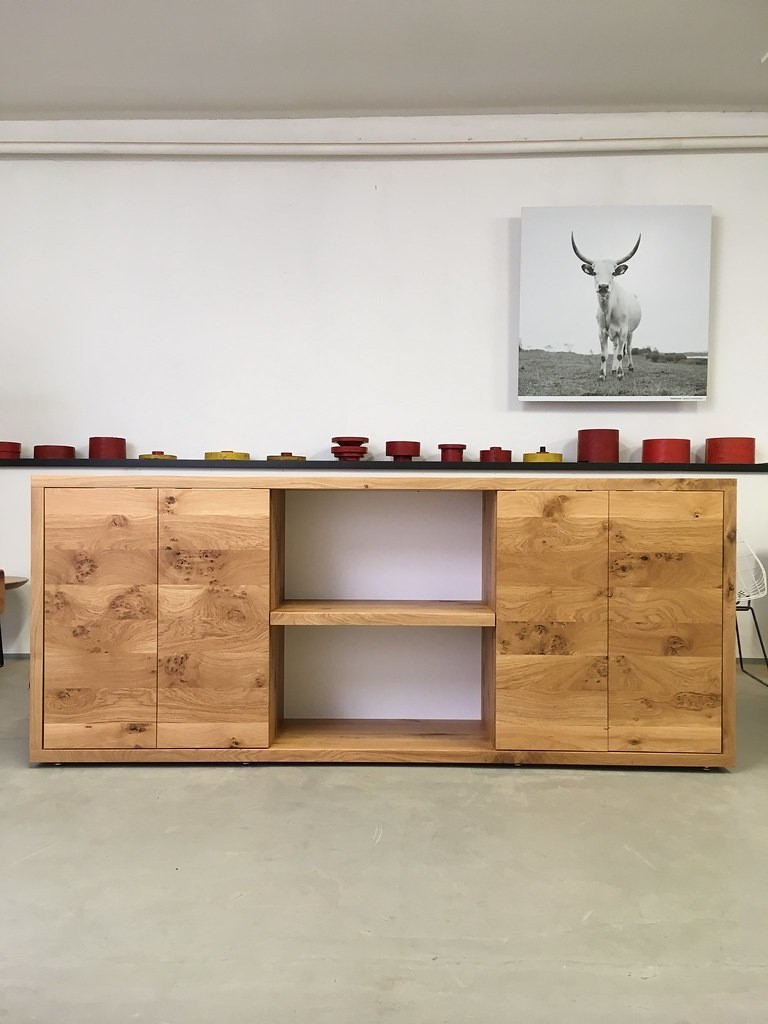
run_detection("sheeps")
[571,230,643,382]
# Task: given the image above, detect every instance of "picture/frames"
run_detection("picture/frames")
[517,205,710,403]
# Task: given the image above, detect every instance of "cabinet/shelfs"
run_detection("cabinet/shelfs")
[30,476,737,772]
